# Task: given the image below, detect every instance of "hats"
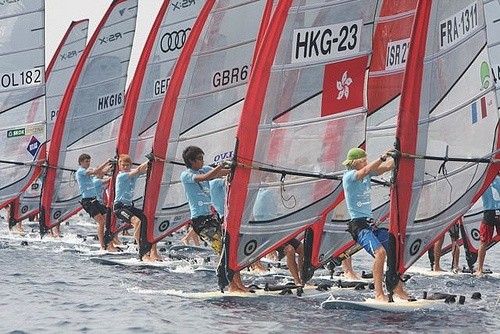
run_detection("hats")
[342,148,367,165]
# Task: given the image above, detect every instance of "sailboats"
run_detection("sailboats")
[0,0,500,312]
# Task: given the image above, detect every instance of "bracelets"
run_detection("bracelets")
[380,155,386,162]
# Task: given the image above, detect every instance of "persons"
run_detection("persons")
[475,170,500,276]
[342,148,410,301]
[3,146,360,294]
[433,220,462,272]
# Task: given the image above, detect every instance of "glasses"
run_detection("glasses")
[195,156,203,161]
